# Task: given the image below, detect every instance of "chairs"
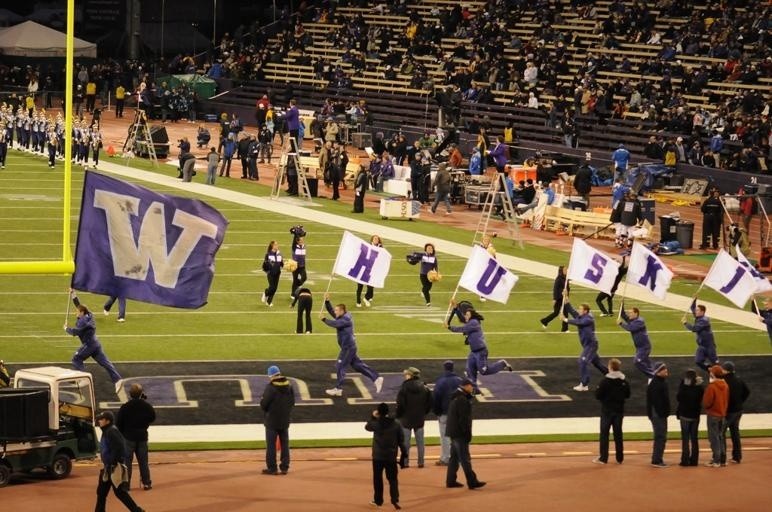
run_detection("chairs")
[544,205,620,241]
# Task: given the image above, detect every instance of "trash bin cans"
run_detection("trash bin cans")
[675,221,695,249]
[658,215,680,243]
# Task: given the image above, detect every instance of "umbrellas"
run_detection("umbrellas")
[0,20,97,58]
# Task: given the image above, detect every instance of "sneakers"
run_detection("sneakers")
[370,499,382,506]
[306,331,310,334]
[615,460,624,464]
[325,388,343,395]
[103,309,110,315]
[564,330,569,332]
[654,464,669,467]
[363,297,370,307]
[728,458,736,462]
[480,297,485,302]
[704,460,720,466]
[573,381,588,390]
[608,314,613,316]
[374,377,383,393]
[291,295,295,299]
[393,502,400,509]
[117,318,124,321]
[262,468,276,473]
[427,303,430,306]
[600,313,607,316]
[720,462,727,465]
[262,292,266,303]
[281,469,286,473]
[592,458,605,464]
[369,297,372,301]
[428,208,433,213]
[266,301,273,307]
[446,212,450,214]
[436,459,447,466]
[647,377,652,384]
[144,485,150,489]
[503,359,513,372]
[420,290,425,298]
[542,324,546,329]
[355,302,361,307]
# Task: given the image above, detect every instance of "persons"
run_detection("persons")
[354,234,382,309]
[64,288,122,384]
[681,293,719,369]
[419,243,438,306]
[206,147,221,185]
[646,361,671,469]
[676,369,705,466]
[700,361,728,468]
[749,296,772,346]
[290,236,307,300]
[103,295,126,322]
[446,379,487,489]
[559,290,609,392]
[116,383,157,491]
[319,292,384,397]
[592,358,631,462]
[179,152,196,182]
[0,59,103,169]
[238,1,384,212]
[261,365,295,475]
[596,256,628,318]
[396,367,432,468]
[179,136,190,154]
[103,38,237,122]
[719,361,751,464]
[220,113,230,137]
[218,133,236,178]
[442,300,513,393]
[196,127,211,147]
[383,0,772,243]
[479,236,496,302]
[616,298,655,385]
[290,288,313,335]
[95,410,145,512]
[260,241,283,307]
[365,403,406,510]
[431,361,464,466]
[759,247,772,273]
[539,265,570,333]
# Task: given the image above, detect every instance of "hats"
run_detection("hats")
[708,365,721,375]
[416,153,422,158]
[654,363,666,373]
[96,411,114,420]
[721,361,734,371]
[444,360,453,371]
[498,136,504,143]
[378,403,388,415]
[268,365,280,377]
[678,137,683,141]
[462,377,478,387]
[438,161,448,169]
[259,104,264,107]
[405,367,421,378]
[473,148,479,152]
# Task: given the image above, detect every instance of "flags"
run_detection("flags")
[705,248,757,309]
[70,172,228,309]
[568,237,621,296]
[331,231,392,289]
[626,240,674,301]
[736,244,772,295]
[458,244,519,305]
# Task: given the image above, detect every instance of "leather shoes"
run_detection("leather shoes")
[447,480,464,487]
[469,481,486,489]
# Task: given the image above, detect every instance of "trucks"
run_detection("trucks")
[0,362,101,485]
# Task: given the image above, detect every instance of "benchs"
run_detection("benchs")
[571,215,582,222]
[280,155,360,189]
